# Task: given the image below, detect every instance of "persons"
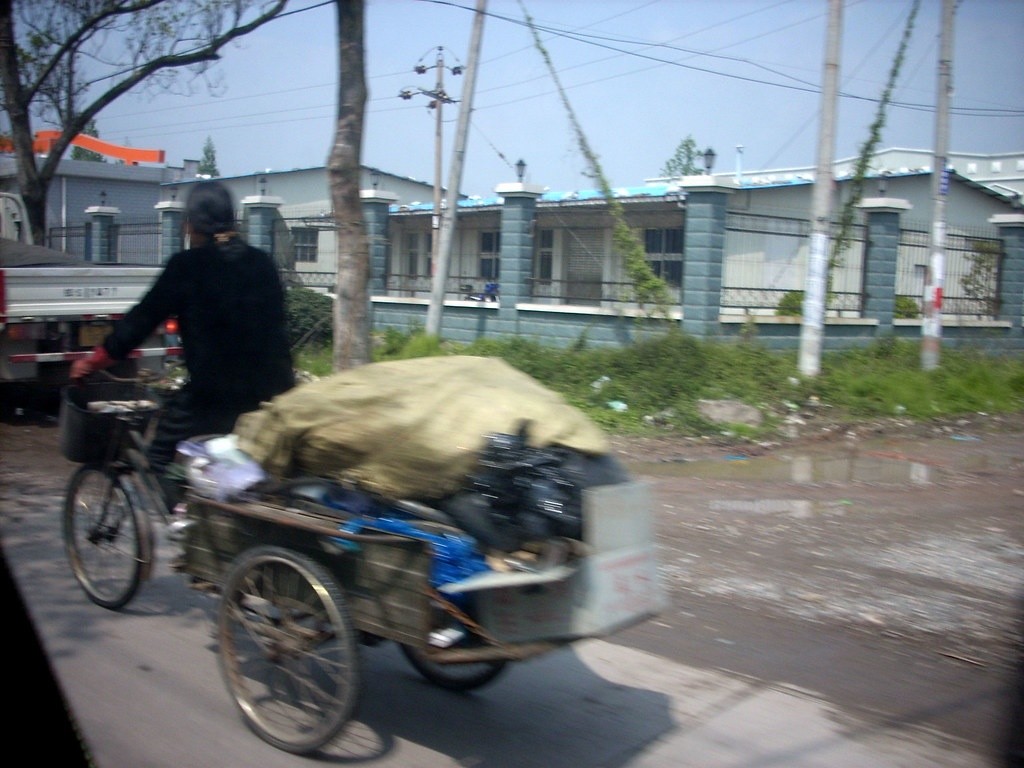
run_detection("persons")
[70,181,300,590]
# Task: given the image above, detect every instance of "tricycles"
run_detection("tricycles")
[58,350,672,758]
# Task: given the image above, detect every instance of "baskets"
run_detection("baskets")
[59,378,155,466]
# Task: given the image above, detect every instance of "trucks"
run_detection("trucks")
[0,190,169,419]
